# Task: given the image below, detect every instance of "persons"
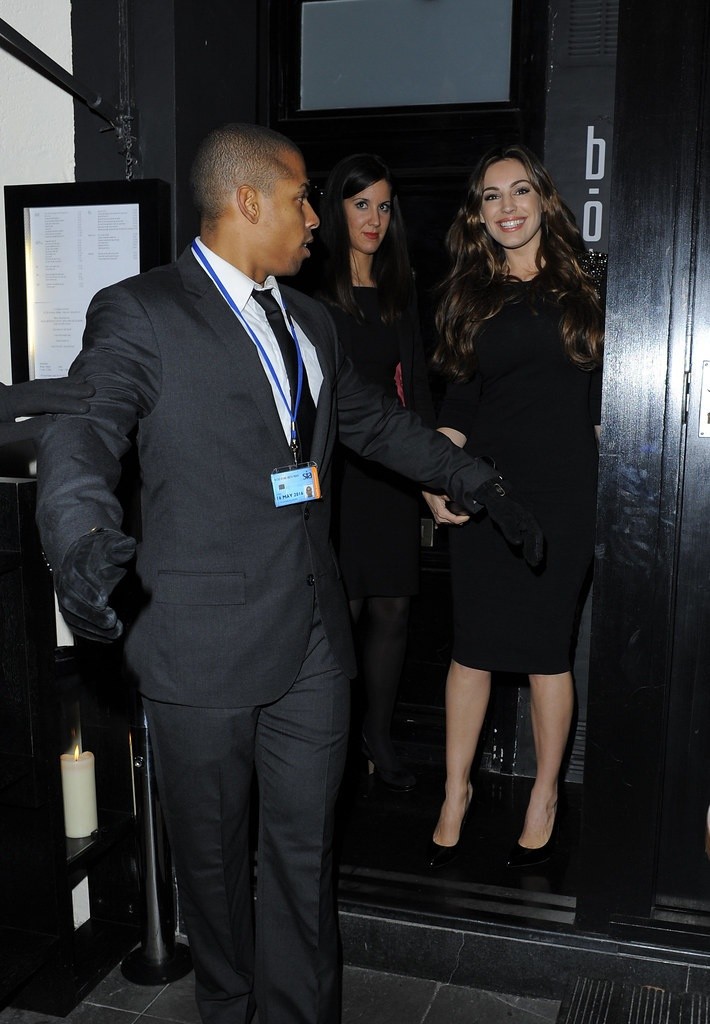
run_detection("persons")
[0,375,96,447]
[306,486,313,497]
[35,120,544,1023]
[307,140,608,872]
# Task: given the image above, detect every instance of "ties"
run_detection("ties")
[251,289,317,469]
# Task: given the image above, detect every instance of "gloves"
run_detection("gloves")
[0,375,95,446]
[472,476,543,569]
[54,531,137,644]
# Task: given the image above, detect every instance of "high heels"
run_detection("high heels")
[361,728,416,793]
[505,793,564,869]
[425,781,478,872]
